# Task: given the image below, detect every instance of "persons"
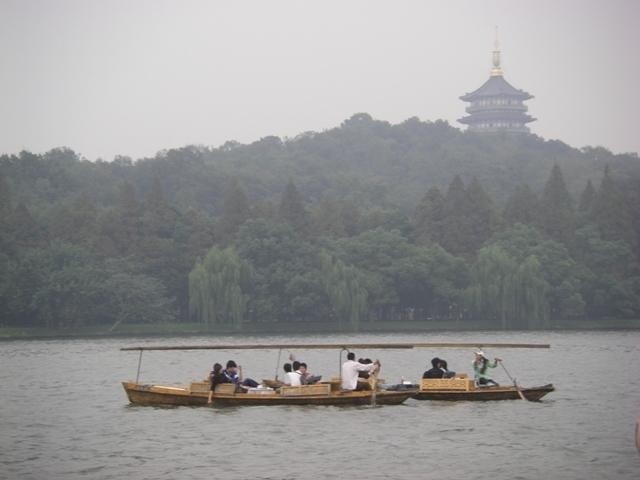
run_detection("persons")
[423,357,448,378]
[208,360,264,404]
[341,353,381,391]
[472,351,503,387]
[280,361,314,386]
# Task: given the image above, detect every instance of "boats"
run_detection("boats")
[115,343,420,408]
[405,341,556,403]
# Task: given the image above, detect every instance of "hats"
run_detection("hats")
[474,351,487,361]
